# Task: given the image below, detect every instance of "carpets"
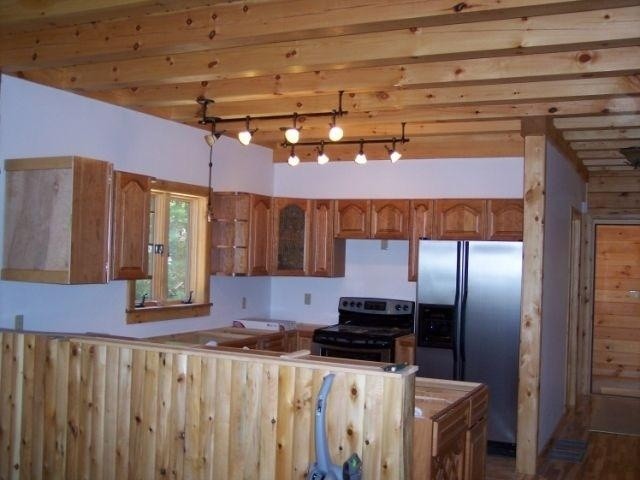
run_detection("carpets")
[590,396,640,438]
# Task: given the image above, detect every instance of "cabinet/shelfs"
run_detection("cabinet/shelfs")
[213,190,346,279]
[333,197,526,242]
[415,375,488,480]
[220,322,325,351]
[112,170,152,280]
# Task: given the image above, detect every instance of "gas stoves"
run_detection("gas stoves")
[318,323,408,340]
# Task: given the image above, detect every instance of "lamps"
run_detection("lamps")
[195,91,349,145]
[280,121,410,167]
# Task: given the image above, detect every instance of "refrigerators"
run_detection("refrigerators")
[415,234,523,458]
[313,343,394,363]
